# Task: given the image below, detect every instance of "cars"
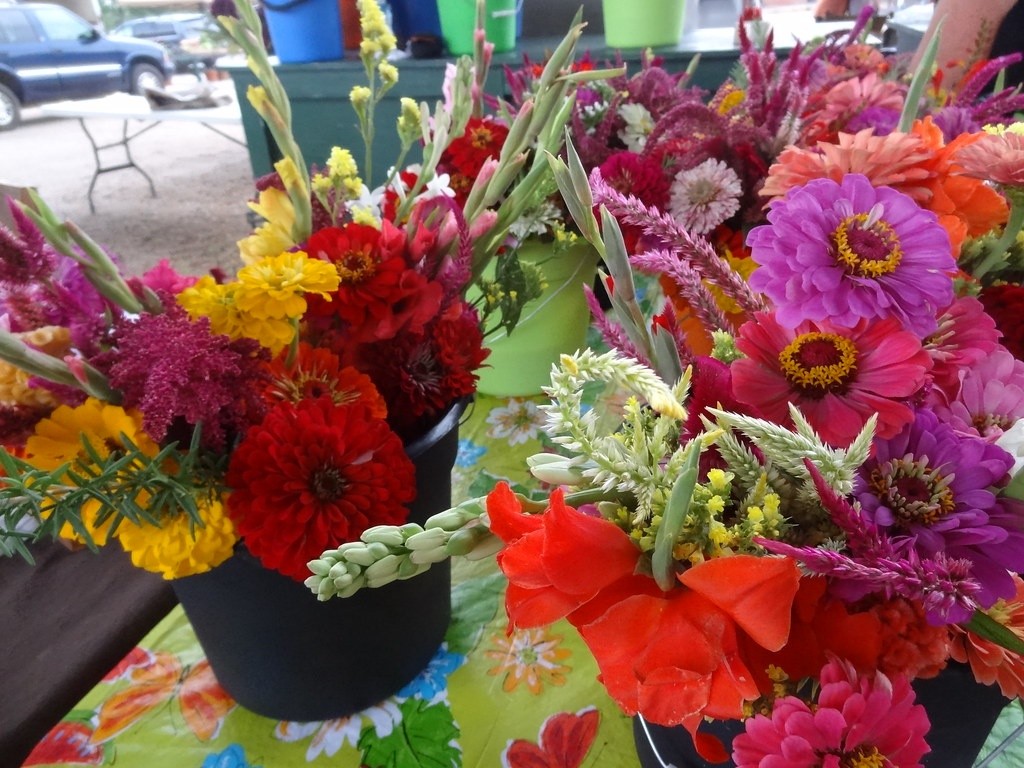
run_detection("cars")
[0,3,175,133]
[110,12,233,68]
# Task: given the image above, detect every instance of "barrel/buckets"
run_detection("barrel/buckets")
[385,0,445,52]
[437,0,518,55]
[258,0,361,64]
[602,0,688,49]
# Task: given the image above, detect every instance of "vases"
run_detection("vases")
[633,653,1014,768]
[162,391,468,721]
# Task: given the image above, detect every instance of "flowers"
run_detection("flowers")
[0,0,1024,768]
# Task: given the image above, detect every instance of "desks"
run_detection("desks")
[214,17,889,191]
[37,82,241,217]
